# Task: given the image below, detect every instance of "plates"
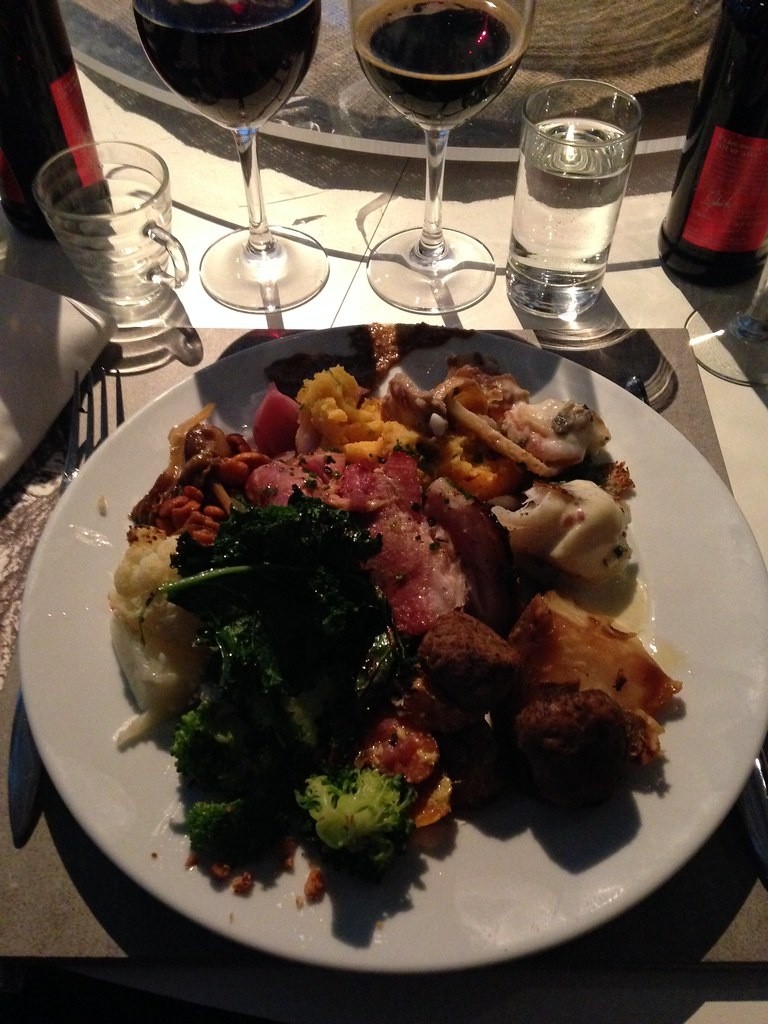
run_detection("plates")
[21,323,767,972]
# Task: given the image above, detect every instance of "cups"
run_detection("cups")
[506,78,643,319]
[33,140,188,308]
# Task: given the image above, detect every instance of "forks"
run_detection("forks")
[8,367,125,846]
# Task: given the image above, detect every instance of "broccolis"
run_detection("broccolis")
[166,701,416,890]
[157,489,410,755]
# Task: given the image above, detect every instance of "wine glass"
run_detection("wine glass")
[683,252,768,388]
[131,3,331,314]
[346,3,534,314]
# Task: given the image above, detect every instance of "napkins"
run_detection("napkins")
[0,268,121,495]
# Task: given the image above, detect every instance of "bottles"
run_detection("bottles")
[660,1,767,286]
[3,2,115,240]
